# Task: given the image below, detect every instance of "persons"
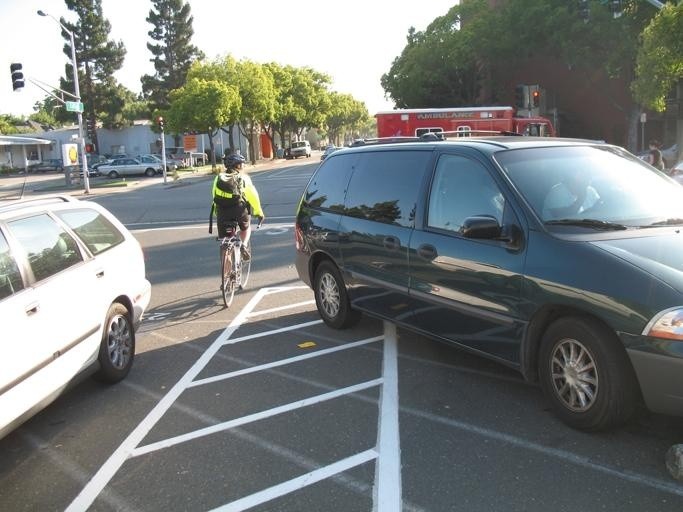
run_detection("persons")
[646,138,664,171]
[206,151,266,290]
[539,159,607,221]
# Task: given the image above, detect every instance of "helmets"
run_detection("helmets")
[224,154,246,169]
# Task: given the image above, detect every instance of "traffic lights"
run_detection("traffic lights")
[10,63,25,90]
[86,143,95,153]
[513,85,523,110]
[533,92,540,107]
[156,117,164,133]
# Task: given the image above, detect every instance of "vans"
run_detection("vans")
[291,140,311,159]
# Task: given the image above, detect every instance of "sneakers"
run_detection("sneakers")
[240,245,251,261]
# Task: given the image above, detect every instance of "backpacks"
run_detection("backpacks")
[213,173,243,208]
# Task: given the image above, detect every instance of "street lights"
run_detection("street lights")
[37,9,90,191]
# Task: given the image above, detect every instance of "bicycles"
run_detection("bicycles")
[217,214,264,308]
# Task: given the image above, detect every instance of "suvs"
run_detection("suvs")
[161,147,208,167]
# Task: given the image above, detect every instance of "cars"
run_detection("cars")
[78,152,185,178]
[28,159,64,173]
[1,193,152,437]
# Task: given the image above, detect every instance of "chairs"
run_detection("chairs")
[39,231,68,274]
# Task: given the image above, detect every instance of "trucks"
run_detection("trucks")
[374,106,555,142]
